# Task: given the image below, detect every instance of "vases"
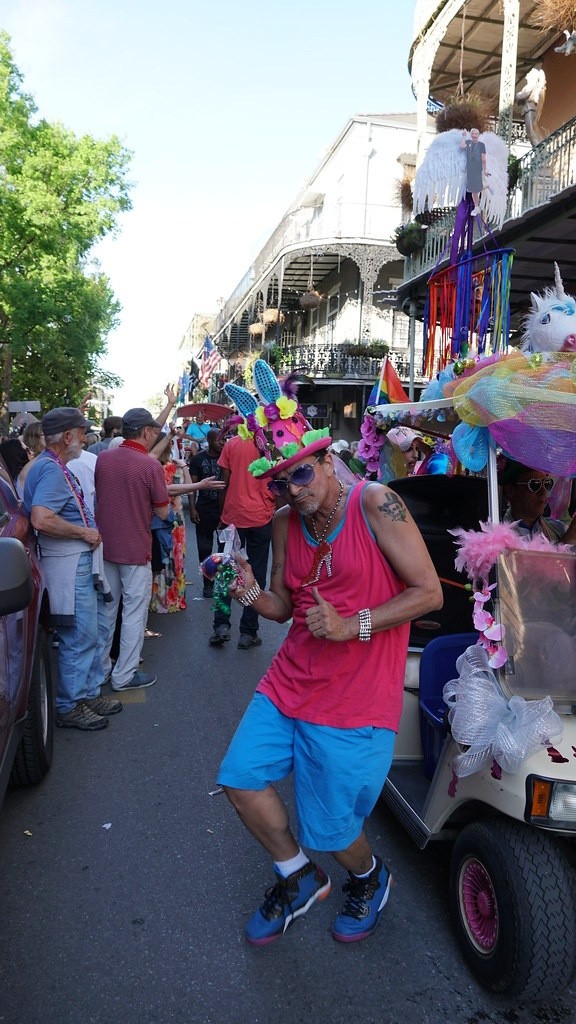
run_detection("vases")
[396,229,426,256]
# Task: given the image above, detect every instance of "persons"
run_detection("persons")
[517,68,542,146]
[94,408,170,690]
[215,450,443,946]
[460,128,494,216]
[502,464,576,636]
[109,383,225,663]
[23,407,123,732]
[0,393,125,508]
[208,435,287,650]
[329,428,451,480]
[84,410,226,613]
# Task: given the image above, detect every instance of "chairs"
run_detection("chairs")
[418,632,483,729]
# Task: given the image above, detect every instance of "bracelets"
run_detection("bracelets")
[237,579,260,606]
[357,608,371,642]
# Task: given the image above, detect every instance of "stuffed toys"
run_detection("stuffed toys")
[517,260,576,354]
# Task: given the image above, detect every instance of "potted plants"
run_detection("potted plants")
[300,291,321,309]
[349,339,390,359]
[236,342,283,384]
[325,370,345,379]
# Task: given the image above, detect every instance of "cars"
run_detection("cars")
[0,456,54,812]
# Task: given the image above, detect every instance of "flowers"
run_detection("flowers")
[473,580,507,671]
[237,396,331,478]
[389,220,422,243]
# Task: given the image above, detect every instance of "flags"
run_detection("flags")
[175,335,223,404]
[367,358,412,407]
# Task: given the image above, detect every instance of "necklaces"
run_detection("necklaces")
[46,449,94,528]
[469,142,477,160]
[301,480,344,587]
[120,444,146,453]
[502,507,563,545]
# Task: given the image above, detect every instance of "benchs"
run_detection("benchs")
[390,475,513,688]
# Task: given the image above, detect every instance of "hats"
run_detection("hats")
[387,426,424,452]
[121,408,162,432]
[224,358,332,478]
[42,408,94,436]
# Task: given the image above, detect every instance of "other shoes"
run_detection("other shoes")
[143,627,161,640]
[202,587,215,598]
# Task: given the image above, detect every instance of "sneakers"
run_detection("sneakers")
[81,693,123,716]
[56,703,109,730]
[329,856,393,943]
[209,621,232,643]
[237,633,263,650]
[114,672,158,691]
[244,860,331,944]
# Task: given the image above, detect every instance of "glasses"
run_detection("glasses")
[504,478,556,493]
[267,455,322,497]
